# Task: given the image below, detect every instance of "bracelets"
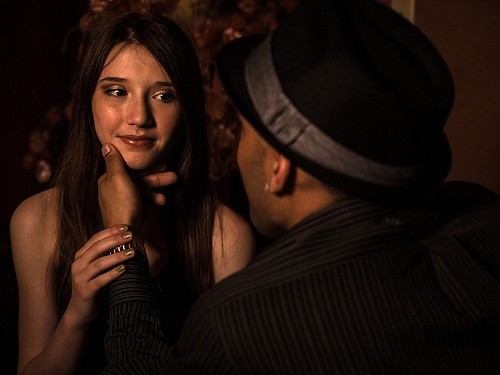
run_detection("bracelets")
[104,233,147,256]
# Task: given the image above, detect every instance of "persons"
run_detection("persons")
[10,12,255,375]
[97,0,500,375]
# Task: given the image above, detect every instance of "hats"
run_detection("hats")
[216,0,456,205]
